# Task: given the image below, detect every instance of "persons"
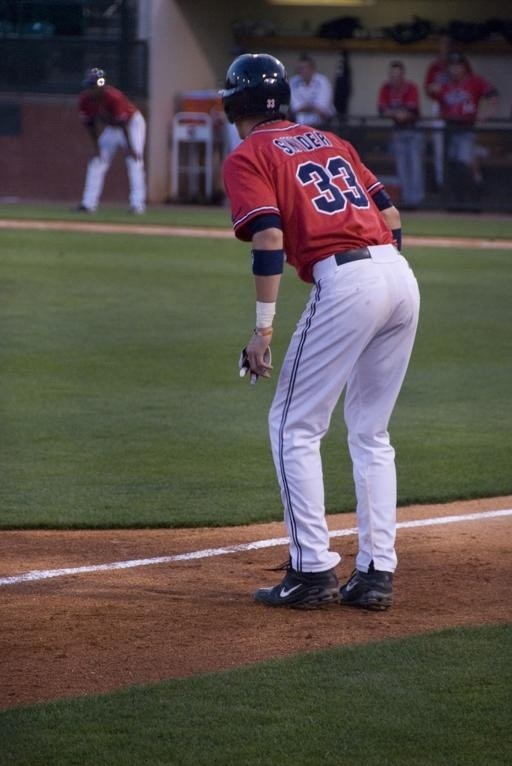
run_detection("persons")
[376,59,430,211]
[425,49,500,211]
[69,67,149,217]
[219,49,422,610]
[286,52,336,133]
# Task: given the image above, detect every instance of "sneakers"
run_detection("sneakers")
[255,567,344,607]
[335,568,393,611]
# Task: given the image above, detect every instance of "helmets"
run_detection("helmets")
[218,53,290,123]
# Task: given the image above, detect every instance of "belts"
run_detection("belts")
[335,246,371,266]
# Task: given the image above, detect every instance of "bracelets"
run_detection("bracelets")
[252,326,276,336]
[254,300,276,330]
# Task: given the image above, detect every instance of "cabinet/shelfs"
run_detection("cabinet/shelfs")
[167,110,226,205]
